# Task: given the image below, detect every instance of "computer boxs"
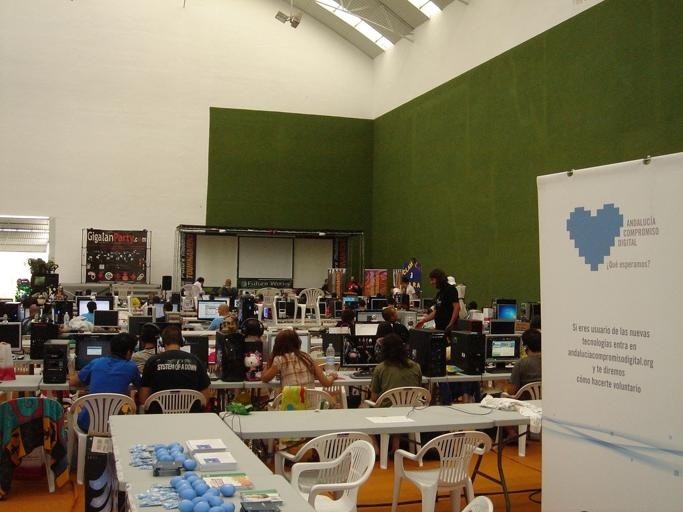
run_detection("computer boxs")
[450,331,485,375]
[215,330,245,382]
[29,323,59,359]
[408,328,446,377]
[43,339,70,384]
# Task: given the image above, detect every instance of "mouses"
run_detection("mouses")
[18,355,24,359]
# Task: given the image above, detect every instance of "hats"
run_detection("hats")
[447,276,455,285]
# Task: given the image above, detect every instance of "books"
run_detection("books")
[184,439,284,512]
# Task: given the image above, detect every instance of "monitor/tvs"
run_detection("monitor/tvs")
[75,334,117,371]
[486,334,520,374]
[340,334,383,379]
[155,336,209,369]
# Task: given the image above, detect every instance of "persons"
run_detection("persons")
[373,336,423,460]
[468,299,540,334]
[131,322,162,372]
[142,324,212,413]
[185,274,270,360]
[322,264,467,334]
[74,334,141,431]
[261,330,338,391]
[21,283,181,337]
[502,329,541,443]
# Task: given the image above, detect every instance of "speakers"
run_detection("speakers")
[45,274,58,287]
[162,276,172,290]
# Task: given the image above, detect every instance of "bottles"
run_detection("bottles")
[63,312,70,329]
[326,343,336,373]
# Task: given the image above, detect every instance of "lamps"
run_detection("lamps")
[316,1,395,52]
[409,0,442,19]
